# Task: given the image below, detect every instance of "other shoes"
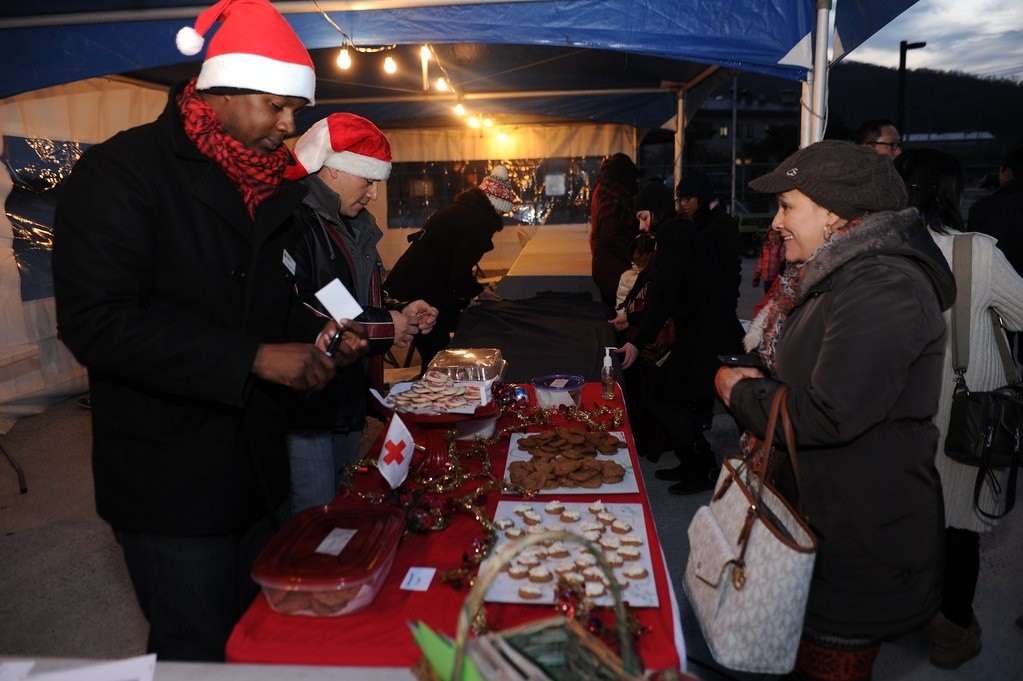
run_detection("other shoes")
[655,463,715,496]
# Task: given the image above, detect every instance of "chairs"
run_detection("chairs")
[472,264,503,291]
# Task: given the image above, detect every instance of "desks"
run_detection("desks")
[224,224,702,681]
[0,343,41,493]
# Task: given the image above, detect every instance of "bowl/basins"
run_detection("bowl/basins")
[531,373,585,410]
[249,502,405,615]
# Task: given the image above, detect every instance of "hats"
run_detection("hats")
[476,166,511,213]
[635,175,669,213]
[285,113,393,181]
[678,167,717,196]
[749,140,908,220]
[175,0,317,107]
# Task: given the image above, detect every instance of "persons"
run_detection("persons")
[913,162,1023,669]
[277,111,437,515]
[382,166,513,378]
[589,152,638,320]
[607,172,747,496]
[752,223,789,294]
[852,118,903,161]
[51,0,367,664]
[716,140,957,681]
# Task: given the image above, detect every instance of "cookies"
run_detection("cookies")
[266,589,358,615]
[508,426,627,489]
[494,499,649,599]
[385,371,481,410]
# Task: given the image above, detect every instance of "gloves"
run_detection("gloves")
[752,277,759,288]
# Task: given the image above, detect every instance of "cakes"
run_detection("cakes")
[426,348,503,384]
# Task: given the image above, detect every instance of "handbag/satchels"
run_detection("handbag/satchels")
[943,374,1023,469]
[683,389,817,675]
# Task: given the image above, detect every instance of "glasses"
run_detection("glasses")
[865,141,902,151]
